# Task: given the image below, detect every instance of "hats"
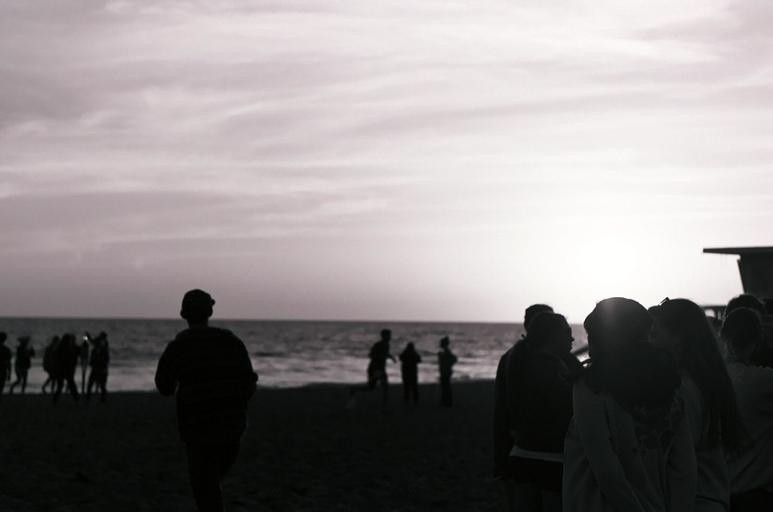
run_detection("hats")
[583,297,654,345]
[181,289,215,316]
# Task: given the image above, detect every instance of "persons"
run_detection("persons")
[487,290,772,511]
[397,342,422,404]
[153,288,260,510]
[350,328,397,407]
[437,335,457,407]
[0,330,115,406]
